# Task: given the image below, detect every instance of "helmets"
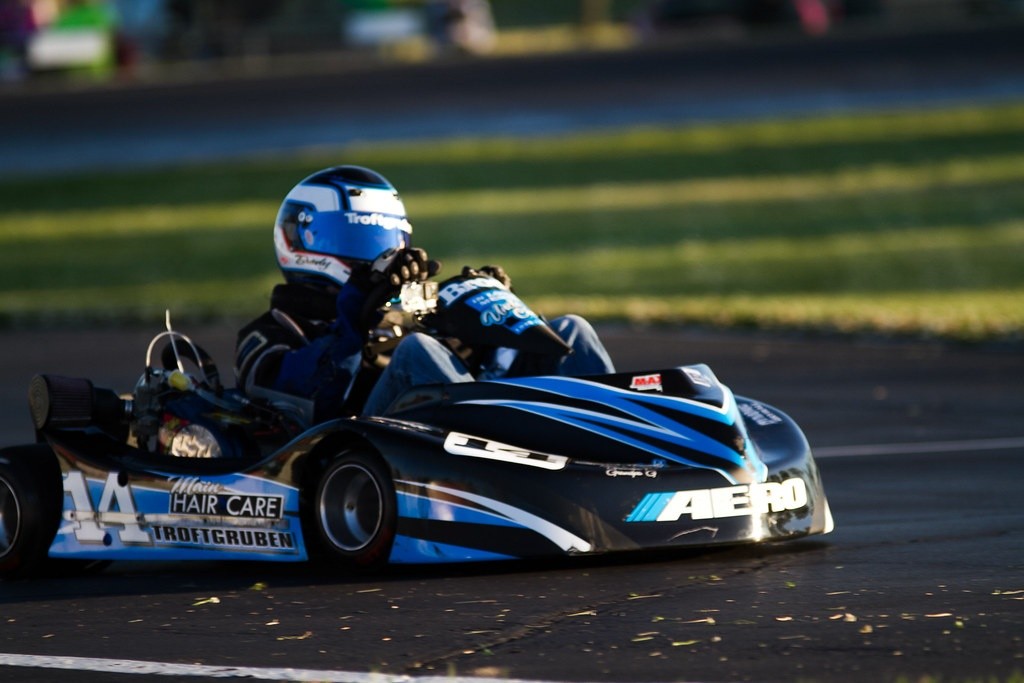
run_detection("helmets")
[273,165,412,297]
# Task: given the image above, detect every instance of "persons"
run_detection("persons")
[237,164,616,418]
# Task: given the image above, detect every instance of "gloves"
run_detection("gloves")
[462,264,513,293]
[348,247,442,308]
